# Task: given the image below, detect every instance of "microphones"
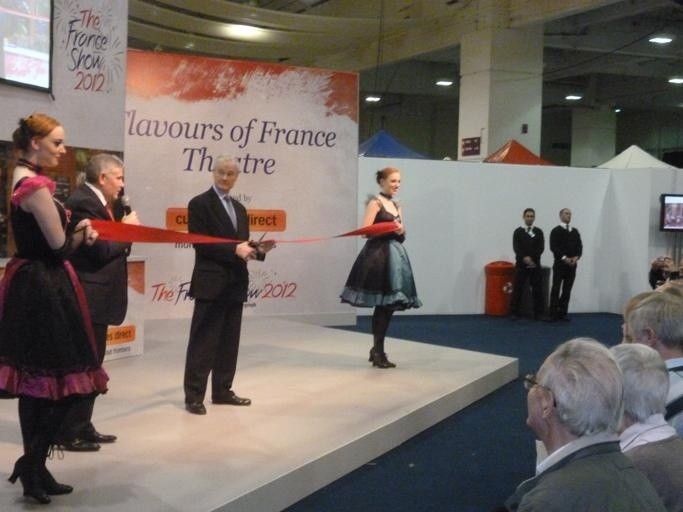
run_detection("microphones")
[121,194,131,215]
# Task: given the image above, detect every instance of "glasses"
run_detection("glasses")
[522,373,557,408]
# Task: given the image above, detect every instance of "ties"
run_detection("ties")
[526,227,531,234]
[106,205,115,223]
[224,197,237,233]
[566,224,570,233]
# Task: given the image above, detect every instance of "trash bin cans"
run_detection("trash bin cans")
[484,261,514,317]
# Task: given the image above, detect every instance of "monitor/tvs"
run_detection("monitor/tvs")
[0,0,55,93]
[659,194,683,231]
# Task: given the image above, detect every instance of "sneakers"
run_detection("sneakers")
[509,314,571,322]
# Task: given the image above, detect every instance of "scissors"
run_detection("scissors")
[248,232,277,249]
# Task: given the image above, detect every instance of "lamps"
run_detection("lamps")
[564,94,584,101]
[365,93,382,103]
[667,74,683,84]
[648,29,677,44]
[434,78,453,87]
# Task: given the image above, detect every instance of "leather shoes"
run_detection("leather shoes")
[87,429,116,444]
[185,401,206,415]
[211,396,251,406]
[56,436,100,452]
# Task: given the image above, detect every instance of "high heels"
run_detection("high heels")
[8,455,50,503]
[368,347,396,369]
[37,462,73,497]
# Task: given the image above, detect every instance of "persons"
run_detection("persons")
[508,206,551,324]
[183,155,275,414]
[339,166,422,369]
[546,208,583,320]
[505,338,666,512]
[0,113,109,502]
[610,344,682,512]
[623,260,683,436]
[55,152,142,452]
